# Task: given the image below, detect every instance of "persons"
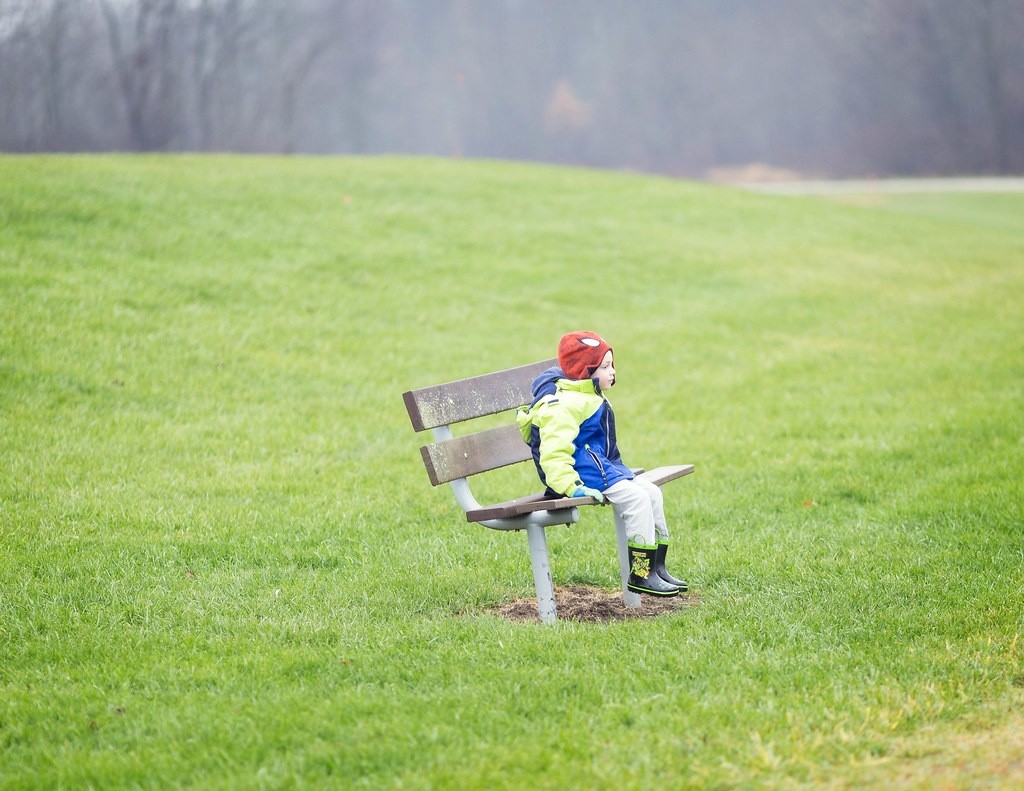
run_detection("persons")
[531,331,688,597]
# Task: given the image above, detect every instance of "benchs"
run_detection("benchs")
[400,352,695,619]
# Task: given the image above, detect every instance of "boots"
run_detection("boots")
[627,535,679,596]
[655,528,689,592]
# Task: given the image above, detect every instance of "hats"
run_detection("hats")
[559,332,617,390]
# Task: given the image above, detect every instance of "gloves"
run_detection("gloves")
[570,486,607,506]
